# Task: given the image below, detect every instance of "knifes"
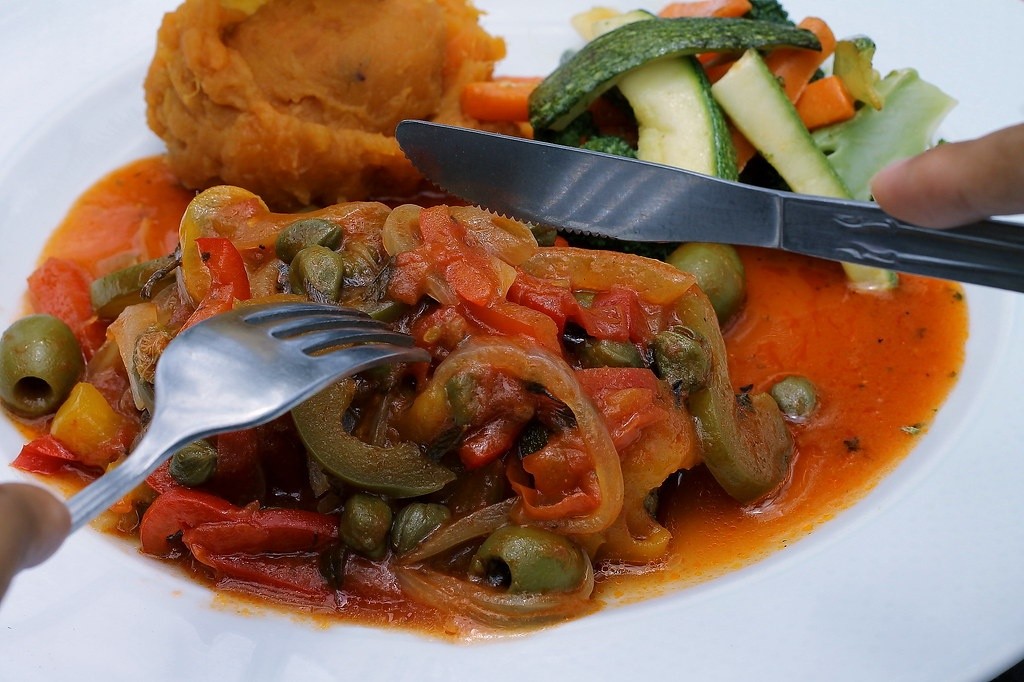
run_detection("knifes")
[394,115,1024,292]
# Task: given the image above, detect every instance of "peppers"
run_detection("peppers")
[15,206,784,623]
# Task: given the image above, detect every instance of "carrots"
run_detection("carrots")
[463,1,859,185]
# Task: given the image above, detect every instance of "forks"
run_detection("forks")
[57,300,430,533]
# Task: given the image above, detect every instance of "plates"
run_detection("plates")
[0,2,1021,679]
